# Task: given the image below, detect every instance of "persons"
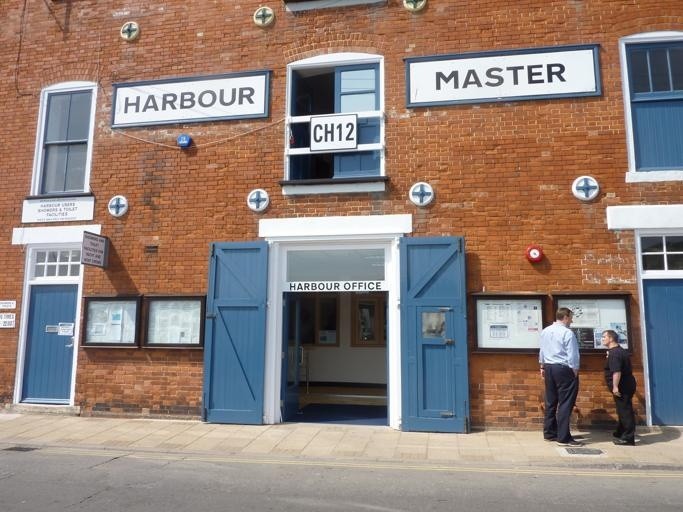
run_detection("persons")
[538,307,581,448]
[600,330,637,446]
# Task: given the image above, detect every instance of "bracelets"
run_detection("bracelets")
[540,368,544,370]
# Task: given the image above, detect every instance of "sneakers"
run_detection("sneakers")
[543,435,585,448]
[612,430,638,448]
